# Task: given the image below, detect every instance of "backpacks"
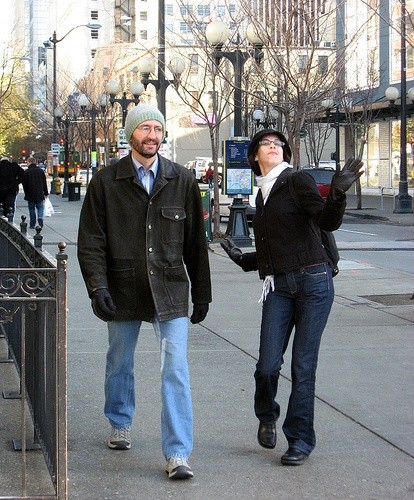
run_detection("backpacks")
[286,171,340,278]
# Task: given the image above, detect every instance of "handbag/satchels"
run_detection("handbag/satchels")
[43,198,54,217]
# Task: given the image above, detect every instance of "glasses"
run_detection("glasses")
[138,124,164,135]
[259,140,284,146]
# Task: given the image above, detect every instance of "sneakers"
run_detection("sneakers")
[108,427,131,450]
[165,457,194,479]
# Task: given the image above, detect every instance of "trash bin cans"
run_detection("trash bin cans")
[201,189,213,243]
[67,182,81,201]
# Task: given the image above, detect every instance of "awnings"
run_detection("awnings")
[299,80,414,122]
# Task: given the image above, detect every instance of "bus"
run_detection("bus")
[44,150,80,178]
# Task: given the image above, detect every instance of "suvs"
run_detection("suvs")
[302,166,336,202]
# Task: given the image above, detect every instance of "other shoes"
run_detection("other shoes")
[38,218,43,227]
[30,224,35,229]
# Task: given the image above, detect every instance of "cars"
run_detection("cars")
[209,174,258,222]
[17,163,46,172]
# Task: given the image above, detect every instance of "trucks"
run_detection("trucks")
[185,157,224,187]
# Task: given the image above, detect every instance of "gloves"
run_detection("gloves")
[220,239,242,265]
[329,157,365,200]
[190,303,209,325]
[91,289,116,322]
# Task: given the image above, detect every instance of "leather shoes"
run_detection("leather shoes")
[281,450,307,465]
[257,422,276,449]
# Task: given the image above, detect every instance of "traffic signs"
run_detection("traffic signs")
[117,128,132,148]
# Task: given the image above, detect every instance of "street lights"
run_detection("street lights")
[322,94,352,172]
[106,78,144,128]
[207,19,270,247]
[79,93,107,175]
[49,24,102,194]
[138,55,185,142]
[385,86,414,214]
[55,106,80,197]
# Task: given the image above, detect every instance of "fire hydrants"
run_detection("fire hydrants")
[54,177,62,195]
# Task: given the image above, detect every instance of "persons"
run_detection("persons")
[0,157,24,221]
[20,158,49,229]
[220,127,367,463]
[77,103,214,479]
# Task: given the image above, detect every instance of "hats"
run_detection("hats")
[124,104,165,147]
[247,129,292,176]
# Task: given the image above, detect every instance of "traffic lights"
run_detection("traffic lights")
[22,150,26,154]
[60,138,63,146]
